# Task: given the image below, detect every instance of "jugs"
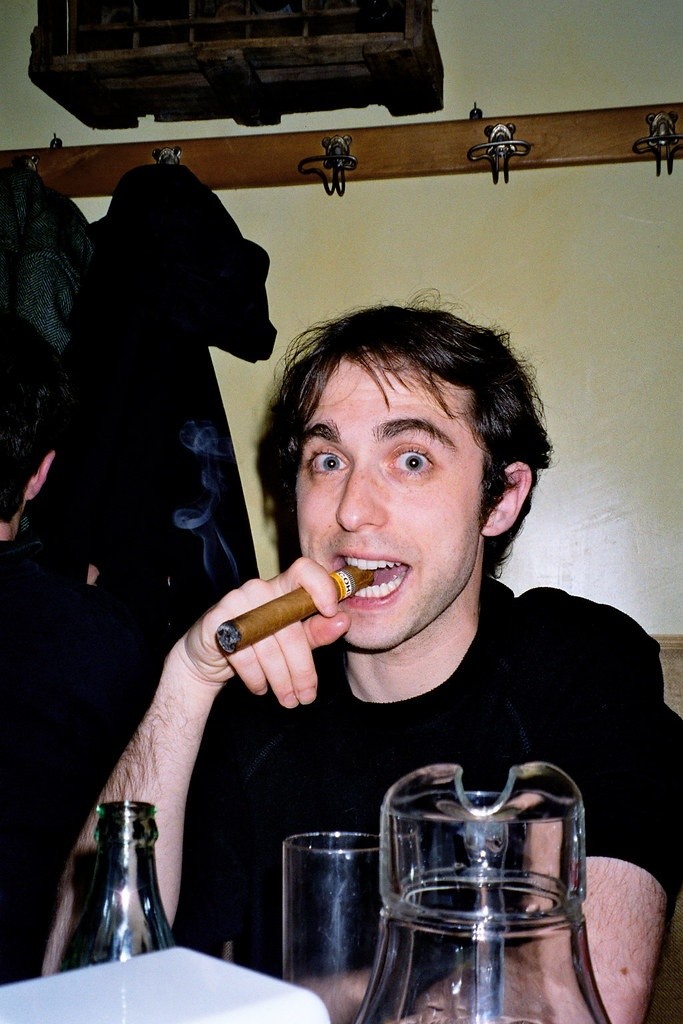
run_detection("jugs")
[353,760,620,1024]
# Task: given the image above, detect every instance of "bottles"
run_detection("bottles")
[53,800,179,978]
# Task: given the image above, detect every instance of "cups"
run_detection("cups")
[279,830,423,1024]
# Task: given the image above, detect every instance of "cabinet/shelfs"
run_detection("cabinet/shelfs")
[27,0,445,131]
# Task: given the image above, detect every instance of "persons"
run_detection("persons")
[1,307,158,986]
[37,306,672,1024]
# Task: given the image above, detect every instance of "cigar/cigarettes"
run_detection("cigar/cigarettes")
[218,565,375,654]
